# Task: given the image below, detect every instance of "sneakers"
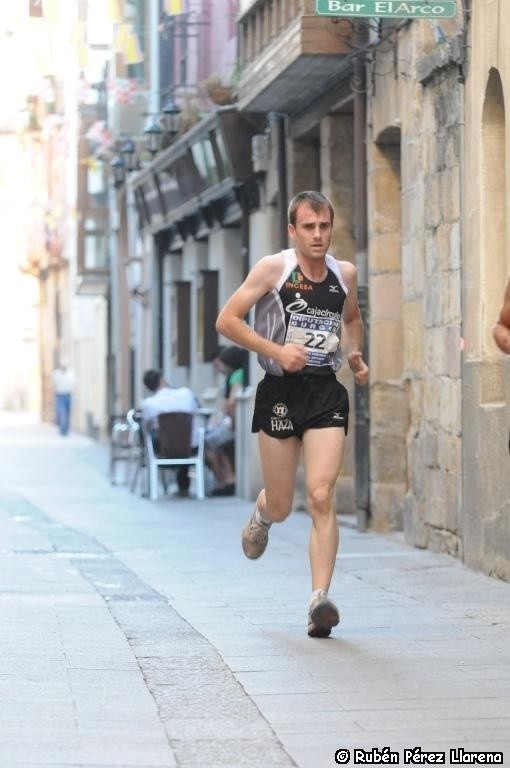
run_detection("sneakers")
[307,590,339,638]
[171,481,235,497]
[242,488,273,560]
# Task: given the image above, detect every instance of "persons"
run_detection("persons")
[144,369,202,499]
[492,273,510,360]
[202,346,247,497]
[205,342,249,399]
[211,188,369,641]
[50,356,79,433]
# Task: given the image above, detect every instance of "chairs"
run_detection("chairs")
[110,409,206,499]
